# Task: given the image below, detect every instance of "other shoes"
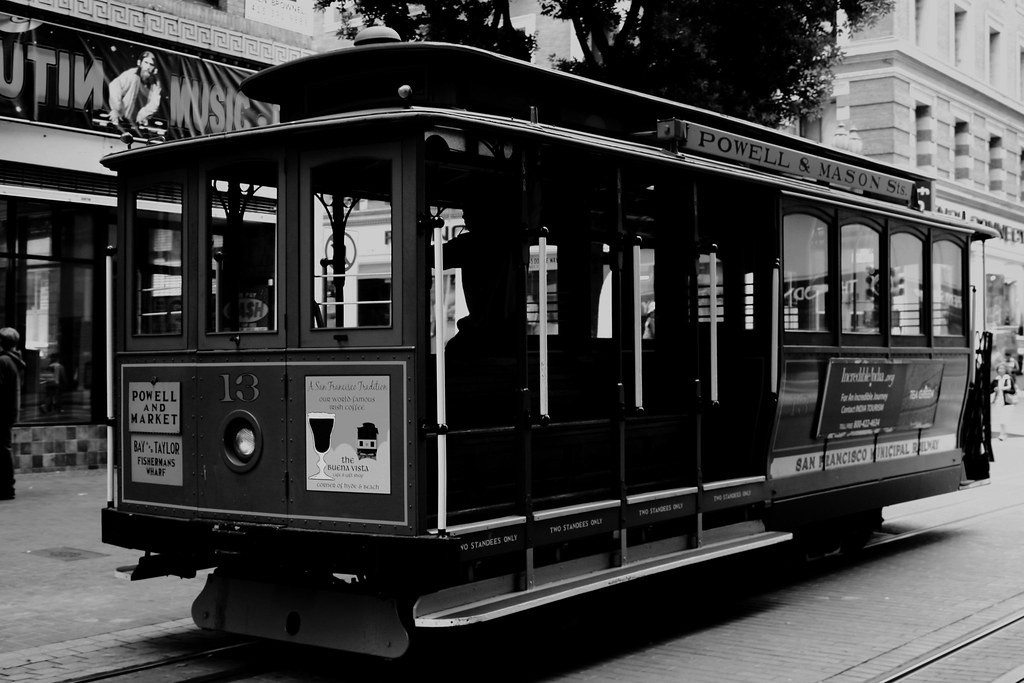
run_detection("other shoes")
[0,487,15,500]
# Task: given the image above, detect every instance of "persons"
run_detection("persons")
[0,326,26,500]
[431,204,528,398]
[38,352,65,414]
[1014,326,1024,376]
[991,364,1016,442]
[108,52,161,126]
[998,351,1019,377]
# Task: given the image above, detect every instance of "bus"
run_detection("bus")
[94,23,1002,658]
